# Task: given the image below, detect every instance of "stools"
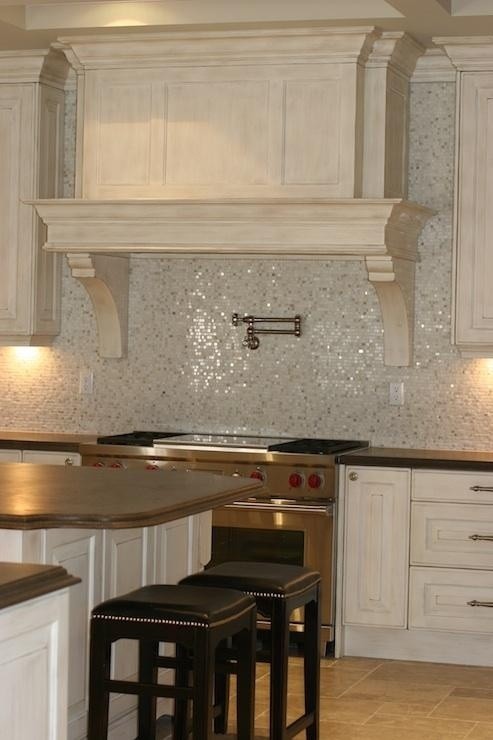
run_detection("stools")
[86,585,257,740]
[198,564,321,738]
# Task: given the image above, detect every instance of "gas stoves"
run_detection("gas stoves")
[78,428,370,505]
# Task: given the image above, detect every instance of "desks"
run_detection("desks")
[1,559,82,740]
[3,465,263,738]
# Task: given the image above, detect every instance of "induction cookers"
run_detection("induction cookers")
[154,434,294,449]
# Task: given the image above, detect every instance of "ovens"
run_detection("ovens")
[206,503,334,659]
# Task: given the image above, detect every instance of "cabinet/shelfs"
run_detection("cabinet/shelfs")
[432,33,492,357]
[0,432,109,466]
[53,24,425,197]
[334,447,412,662]
[1,46,68,345]
[410,451,493,666]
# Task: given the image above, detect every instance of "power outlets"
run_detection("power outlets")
[389,384,403,405]
[79,374,93,393]
